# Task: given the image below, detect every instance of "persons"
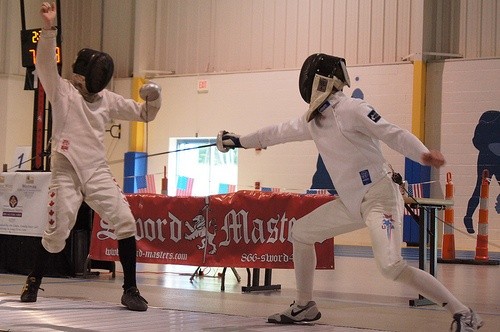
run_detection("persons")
[216,53,486,332]
[20,2,162,311]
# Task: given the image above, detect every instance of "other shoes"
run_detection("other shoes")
[121,285,149,311]
[21,272,44,302]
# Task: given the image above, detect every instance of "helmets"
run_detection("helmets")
[299,53,351,123]
[72,48,115,94]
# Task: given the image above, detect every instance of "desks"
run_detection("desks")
[0,170,454,307]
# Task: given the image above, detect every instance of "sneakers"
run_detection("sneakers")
[268,300,321,324]
[450,306,484,332]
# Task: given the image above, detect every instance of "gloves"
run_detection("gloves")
[222,131,245,149]
